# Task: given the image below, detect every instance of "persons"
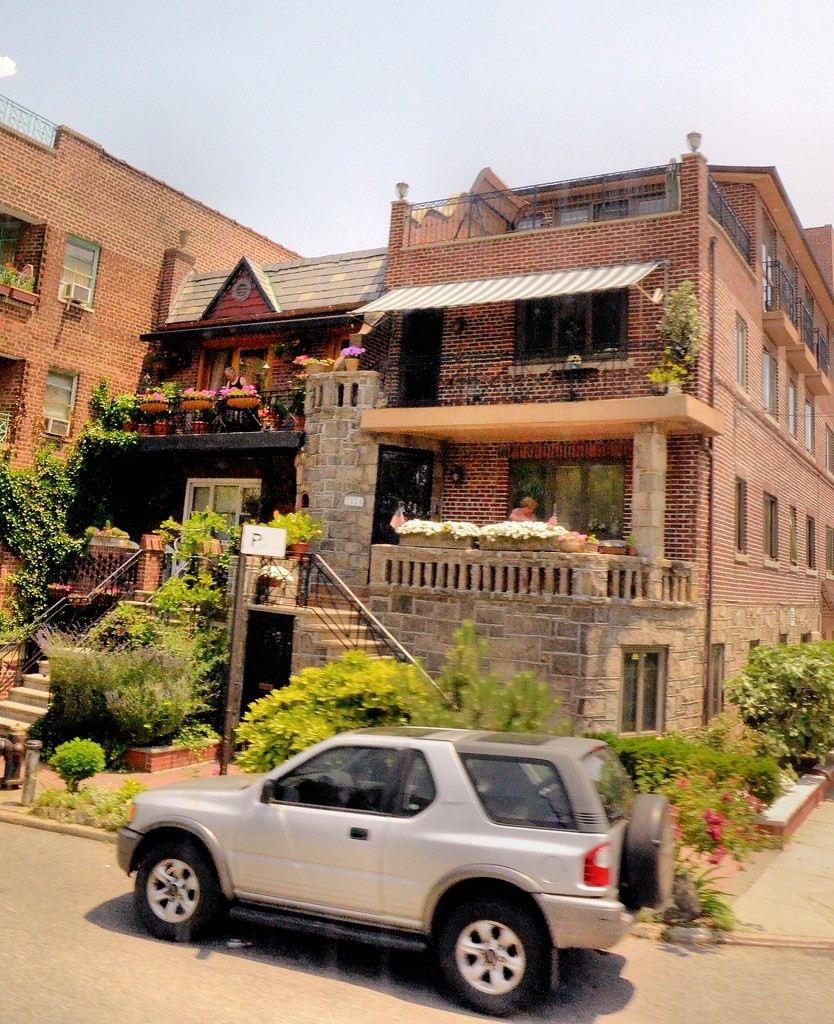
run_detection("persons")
[509,497,538,522]
[222,366,249,392]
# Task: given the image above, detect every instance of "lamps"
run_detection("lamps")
[261,361,270,369]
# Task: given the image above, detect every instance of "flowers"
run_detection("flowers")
[179,388,216,398]
[260,565,294,582]
[558,530,588,544]
[341,346,366,359]
[84,520,129,536]
[479,520,569,542]
[99,586,122,596]
[292,355,335,367]
[218,384,257,399]
[395,518,479,541]
[0,262,18,284]
[68,593,91,608]
[47,582,74,592]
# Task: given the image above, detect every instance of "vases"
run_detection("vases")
[561,541,583,553]
[478,535,561,551]
[181,397,214,411]
[398,532,473,549]
[306,364,325,374]
[85,534,130,546]
[139,400,170,413]
[48,588,72,598]
[137,388,169,403]
[344,358,360,371]
[0,282,11,297]
[104,594,121,603]
[259,577,287,588]
[227,395,261,408]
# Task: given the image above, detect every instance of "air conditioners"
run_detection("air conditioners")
[62,282,91,305]
[43,416,71,437]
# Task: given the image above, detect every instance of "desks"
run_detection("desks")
[551,367,600,401]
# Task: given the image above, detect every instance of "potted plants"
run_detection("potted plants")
[121,413,209,436]
[268,509,327,558]
[258,400,286,431]
[9,273,40,305]
[627,533,637,556]
[289,387,306,431]
[105,682,221,773]
[585,534,599,552]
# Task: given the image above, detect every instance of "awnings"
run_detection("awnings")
[345,259,672,336]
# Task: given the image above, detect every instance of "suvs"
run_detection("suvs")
[117,726,679,1017]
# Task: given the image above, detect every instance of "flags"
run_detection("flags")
[390,506,404,529]
[547,505,558,525]
